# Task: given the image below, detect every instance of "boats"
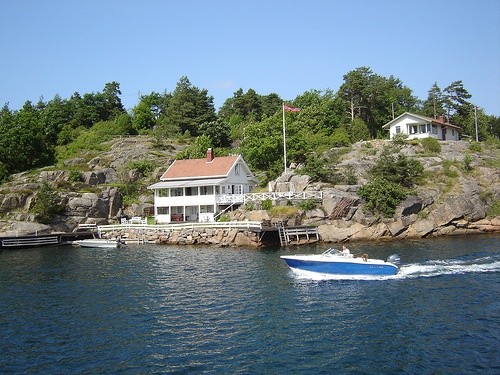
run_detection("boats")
[279,248,401,279]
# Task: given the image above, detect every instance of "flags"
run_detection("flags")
[284,104,301,112]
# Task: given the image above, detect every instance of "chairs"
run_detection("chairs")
[141,216,147,225]
[120,217,128,224]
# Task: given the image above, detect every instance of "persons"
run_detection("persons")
[337,245,351,256]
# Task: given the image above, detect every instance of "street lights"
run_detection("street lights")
[475,106,479,143]
